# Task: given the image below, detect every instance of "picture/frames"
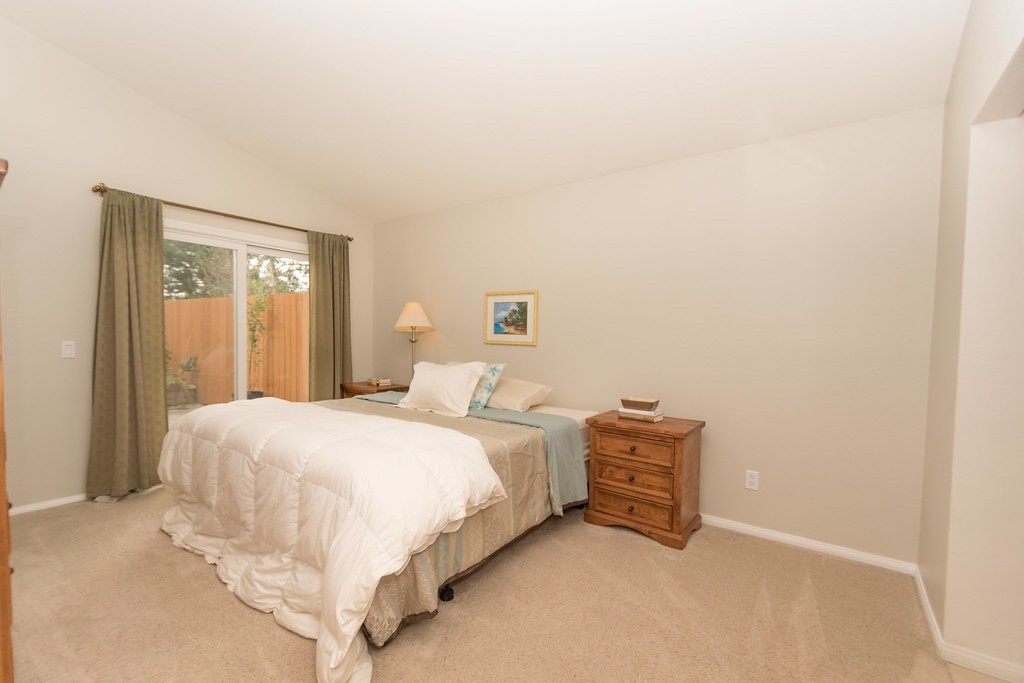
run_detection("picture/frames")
[483,290,538,347]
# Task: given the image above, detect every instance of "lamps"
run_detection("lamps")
[394,302,435,378]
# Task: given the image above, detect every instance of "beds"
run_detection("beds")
[157,390,599,683]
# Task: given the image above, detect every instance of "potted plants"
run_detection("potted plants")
[231,280,270,400]
[164,348,197,405]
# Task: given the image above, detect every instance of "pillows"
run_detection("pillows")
[446,361,506,411]
[392,360,487,418]
[483,376,555,414]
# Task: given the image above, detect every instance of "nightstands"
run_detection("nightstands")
[583,409,707,551]
[340,382,409,399]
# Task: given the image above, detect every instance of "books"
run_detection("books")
[618,408,664,424]
[367,377,392,387]
